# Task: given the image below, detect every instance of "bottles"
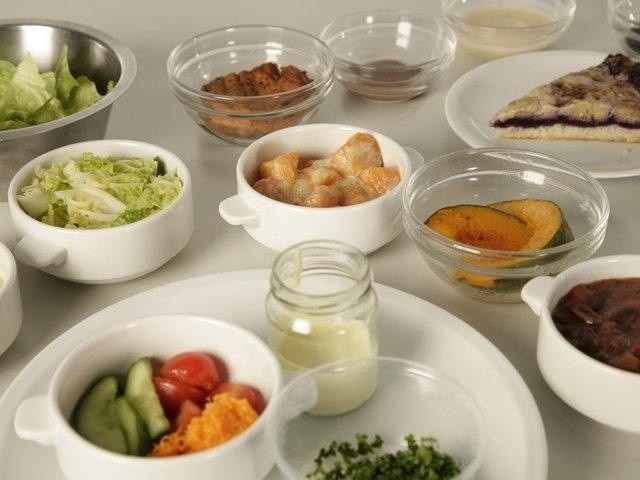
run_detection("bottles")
[267,237,379,417]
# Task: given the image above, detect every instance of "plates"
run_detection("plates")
[444,51,640,177]
[1,274,552,480]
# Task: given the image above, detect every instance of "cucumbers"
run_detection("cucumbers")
[75,356,171,456]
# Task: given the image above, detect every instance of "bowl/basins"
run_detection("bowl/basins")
[9,141,194,287]
[1,13,142,194]
[518,257,640,438]
[16,313,319,477]
[439,1,585,53]
[599,2,640,60]
[162,21,337,132]
[211,121,429,255]
[319,11,457,107]
[400,152,611,312]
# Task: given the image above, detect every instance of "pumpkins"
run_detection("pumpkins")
[421,198,574,290]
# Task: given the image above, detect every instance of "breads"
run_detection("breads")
[488,53,640,143]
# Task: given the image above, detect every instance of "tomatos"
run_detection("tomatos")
[153,352,260,437]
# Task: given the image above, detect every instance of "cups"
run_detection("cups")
[267,357,486,480]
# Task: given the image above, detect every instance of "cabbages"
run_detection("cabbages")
[0,44,113,133]
[17,150,184,230]
